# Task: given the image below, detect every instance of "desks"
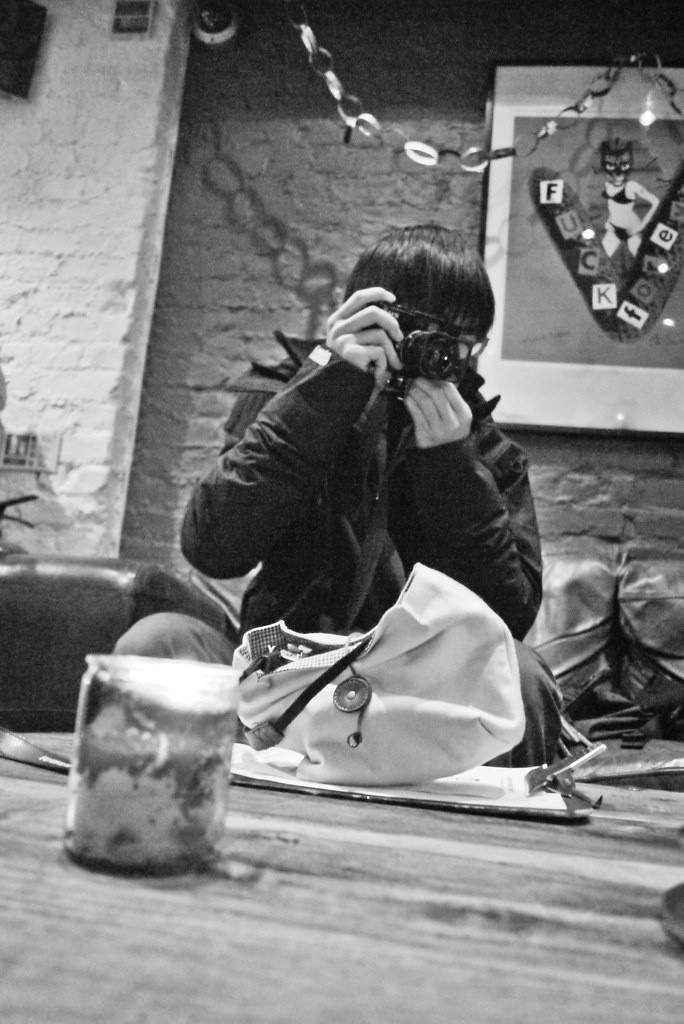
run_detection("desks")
[0,778,684,1024]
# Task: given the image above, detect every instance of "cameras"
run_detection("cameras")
[368,300,461,393]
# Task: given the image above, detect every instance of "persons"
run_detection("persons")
[112,226,563,767]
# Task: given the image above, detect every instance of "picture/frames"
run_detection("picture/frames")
[475,57,684,447]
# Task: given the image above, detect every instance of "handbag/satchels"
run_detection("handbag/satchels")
[232,561,526,787]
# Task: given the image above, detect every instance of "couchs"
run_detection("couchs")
[0,542,684,791]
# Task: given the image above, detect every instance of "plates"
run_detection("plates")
[658,880,684,948]
[676,825,684,851]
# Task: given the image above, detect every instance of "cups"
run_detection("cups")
[61,653,240,881]
